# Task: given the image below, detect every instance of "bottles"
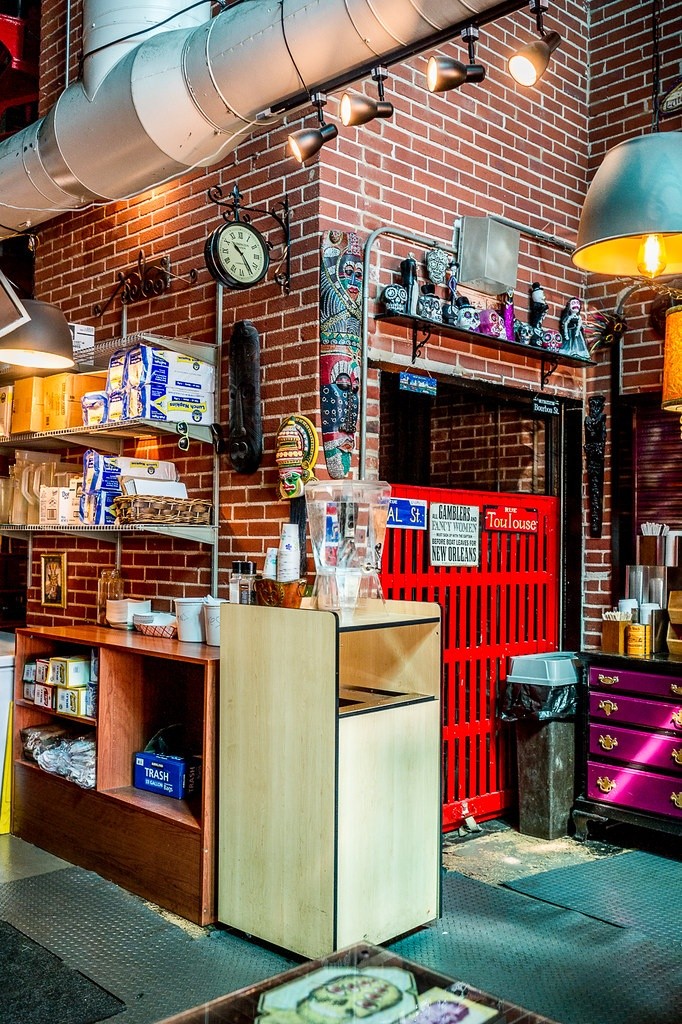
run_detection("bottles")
[229,561,257,605]
[97,569,124,626]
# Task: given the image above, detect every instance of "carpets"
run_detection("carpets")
[498,848,682,945]
[378,870,682,1024]
[0,865,304,1024]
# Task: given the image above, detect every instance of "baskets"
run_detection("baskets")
[114,494,213,525]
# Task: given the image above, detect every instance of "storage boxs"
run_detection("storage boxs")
[23,681,36,702]
[133,723,203,800]
[23,662,36,683]
[57,685,87,716]
[42,372,106,432]
[89,647,99,685]
[36,658,57,687]
[10,376,44,435]
[0,385,13,439]
[46,654,90,688]
[601,620,633,655]
[34,682,56,711]
[86,681,97,718]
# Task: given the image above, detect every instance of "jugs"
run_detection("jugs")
[21,461,51,524]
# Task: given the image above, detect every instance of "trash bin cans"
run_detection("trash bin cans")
[496,652,589,840]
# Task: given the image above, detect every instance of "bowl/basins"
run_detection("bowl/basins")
[174,598,226,642]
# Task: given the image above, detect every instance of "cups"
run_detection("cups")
[261,524,300,583]
[204,605,221,646]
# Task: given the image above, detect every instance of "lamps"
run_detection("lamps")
[289,91,339,164]
[0,298,75,369]
[427,20,486,93]
[660,304,682,413]
[571,0,682,281]
[507,0,562,87]
[339,64,395,127]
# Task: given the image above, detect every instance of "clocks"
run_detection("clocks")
[203,215,270,291]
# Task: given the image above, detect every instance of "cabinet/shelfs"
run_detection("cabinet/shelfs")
[9,625,223,926]
[216,598,445,963]
[0,282,224,600]
[570,650,682,842]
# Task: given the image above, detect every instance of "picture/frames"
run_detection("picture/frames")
[40,551,68,609]
